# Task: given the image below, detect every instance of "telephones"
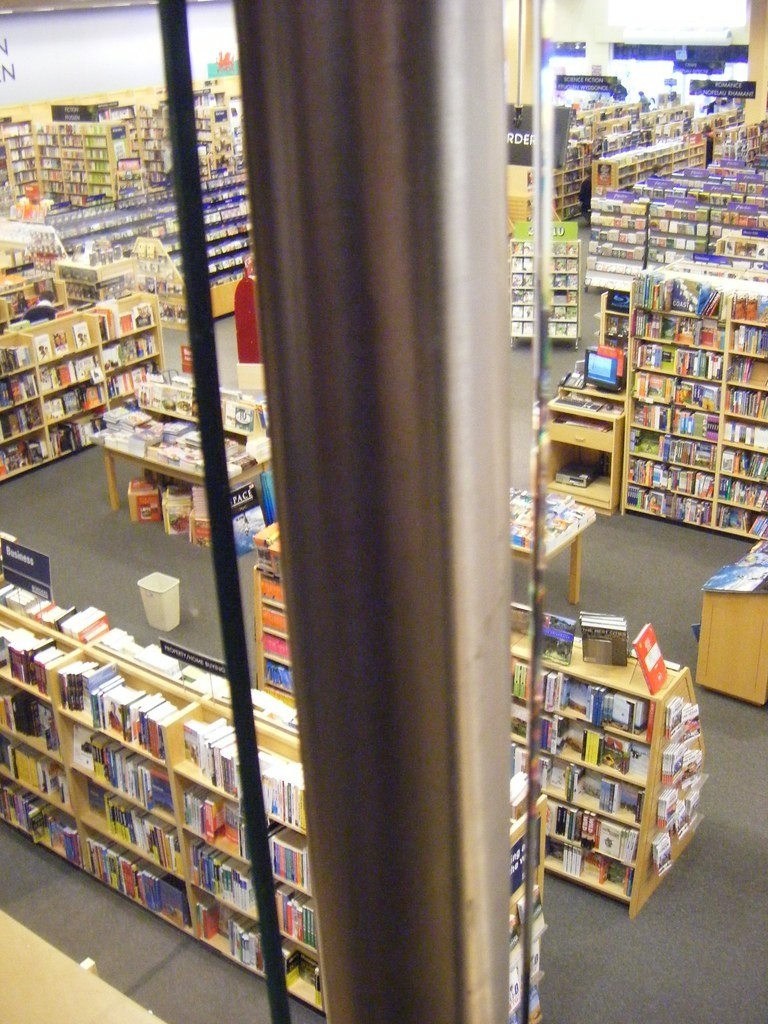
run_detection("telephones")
[560,371,587,389]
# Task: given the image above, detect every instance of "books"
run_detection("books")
[510,487,596,553]
[0,576,542,1024]
[703,541,768,592]
[0,284,283,572]
[1,103,184,275]
[512,244,578,338]
[564,104,766,273]
[193,91,245,177]
[627,273,767,538]
[201,180,247,288]
[510,598,703,890]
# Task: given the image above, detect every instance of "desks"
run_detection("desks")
[90,382,272,513]
[510,487,598,604]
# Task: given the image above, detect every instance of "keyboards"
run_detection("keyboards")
[555,396,602,413]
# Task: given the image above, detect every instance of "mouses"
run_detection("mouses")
[606,404,613,410]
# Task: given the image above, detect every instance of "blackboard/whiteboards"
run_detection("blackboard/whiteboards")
[137,571,182,633]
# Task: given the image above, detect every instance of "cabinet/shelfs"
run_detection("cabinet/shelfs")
[253,522,710,919]
[547,382,626,517]
[621,257,768,542]
[47,628,201,940]
[1,602,86,871]
[1,290,165,483]
[163,681,549,1024]
[0,72,768,388]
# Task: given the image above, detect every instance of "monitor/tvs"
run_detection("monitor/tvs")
[584,349,627,394]
[507,104,571,167]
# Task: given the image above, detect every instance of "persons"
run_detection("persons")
[610,80,627,101]
[702,126,712,164]
[579,167,591,225]
[22,290,58,320]
[639,91,649,112]
[707,97,727,114]
